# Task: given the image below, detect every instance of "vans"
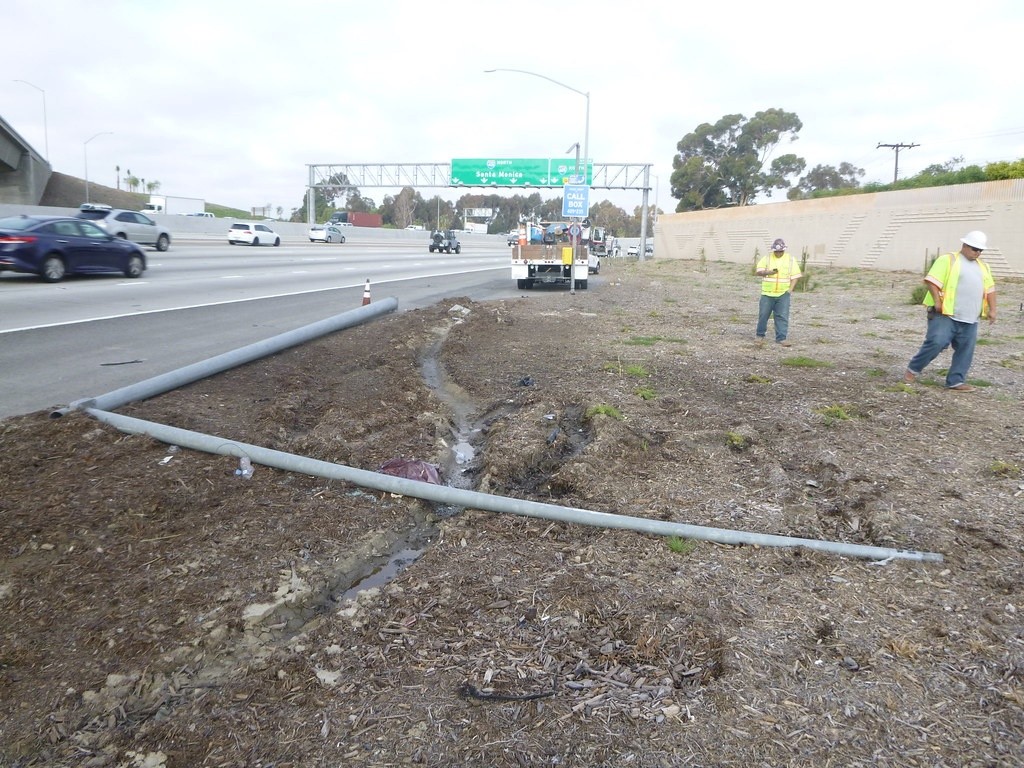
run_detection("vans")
[404,225,422,230]
[309,225,345,243]
[227,223,281,247]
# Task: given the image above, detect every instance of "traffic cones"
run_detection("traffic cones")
[362,279,371,305]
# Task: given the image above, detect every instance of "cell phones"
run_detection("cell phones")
[773,269,777,271]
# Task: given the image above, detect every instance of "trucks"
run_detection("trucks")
[461,223,487,234]
[507,221,621,289]
[139,195,205,214]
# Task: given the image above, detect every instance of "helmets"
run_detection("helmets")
[771,239,786,252]
[960,231,988,250]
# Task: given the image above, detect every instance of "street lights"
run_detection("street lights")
[483,67,590,185]
[83,130,115,203]
[13,79,49,161]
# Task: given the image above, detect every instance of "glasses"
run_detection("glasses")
[968,245,983,252]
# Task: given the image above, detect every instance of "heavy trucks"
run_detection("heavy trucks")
[327,211,383,227]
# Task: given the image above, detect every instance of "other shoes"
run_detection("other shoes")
[754,336,763,342]
[904,370,914,381]
[950,385,975,393]
[777,340,792,346]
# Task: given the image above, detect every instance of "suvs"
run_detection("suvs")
[199,213,215,217]
[429,228,461,254]
[74,209,172,251]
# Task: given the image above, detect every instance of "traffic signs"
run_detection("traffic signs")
[450,158,593,187]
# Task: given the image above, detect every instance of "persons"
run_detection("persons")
[754,239,801,347]
[905,231,997,392]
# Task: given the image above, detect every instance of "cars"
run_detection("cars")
[0,214,147,283]
[263,219,277,222]
[627,245,639,255]
[224,217,237,219]
[80,203,112,209]
